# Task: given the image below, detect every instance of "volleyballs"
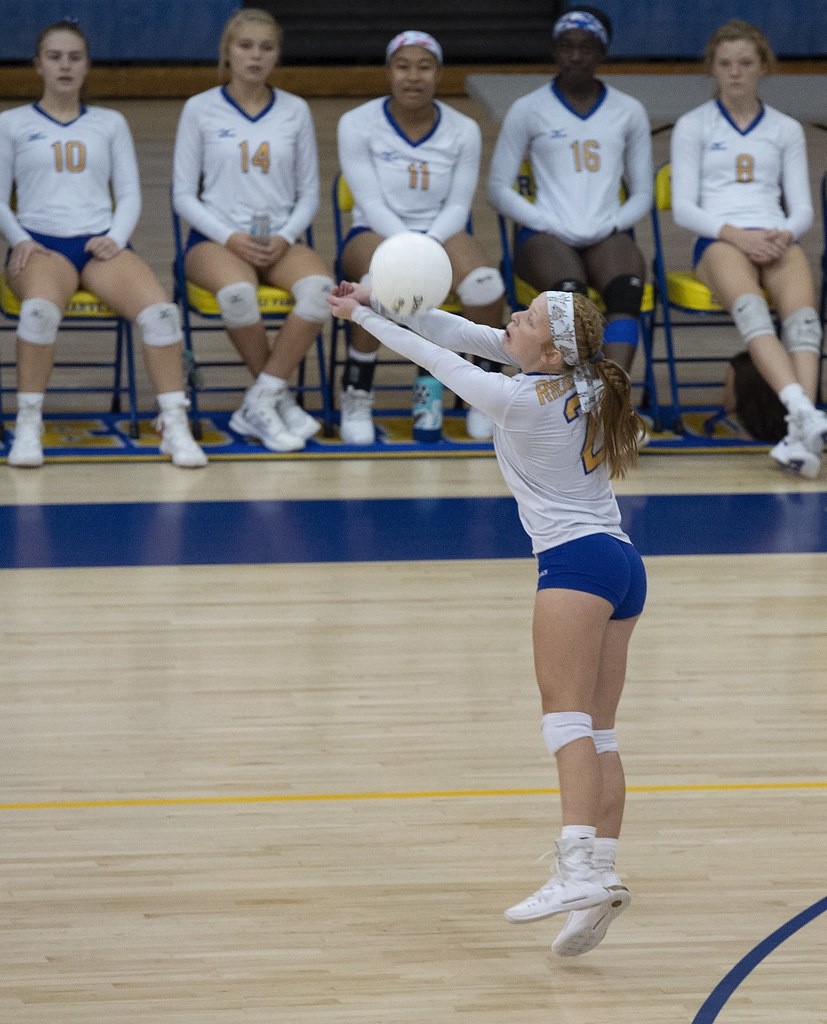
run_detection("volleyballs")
[367,230,452,324]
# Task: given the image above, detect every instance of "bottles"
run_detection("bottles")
[412,365,452,443]
[252,211,272,243]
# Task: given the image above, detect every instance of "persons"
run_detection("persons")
[336,29,504,445]
[0,18,209,469]
[327,280,646,959]
[487,9,654,449]
[172,9,333,453]
[672,18,827,477]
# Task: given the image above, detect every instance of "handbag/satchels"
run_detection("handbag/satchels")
[729,351,787,442]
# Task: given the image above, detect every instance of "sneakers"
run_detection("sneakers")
[505,839,610,924]
[156,398,209,467]
[339,384,375,445]
[769,399,827,481]
[551,871,632,957]
[228,383,322,451]
[467,404,494,439]
[7,399,46,466]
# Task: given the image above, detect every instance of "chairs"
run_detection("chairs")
[497,157,663,438]
[171,179,331,438]
[335,170,476,425]
[654,160,779,437]
[0,227,141,442]
